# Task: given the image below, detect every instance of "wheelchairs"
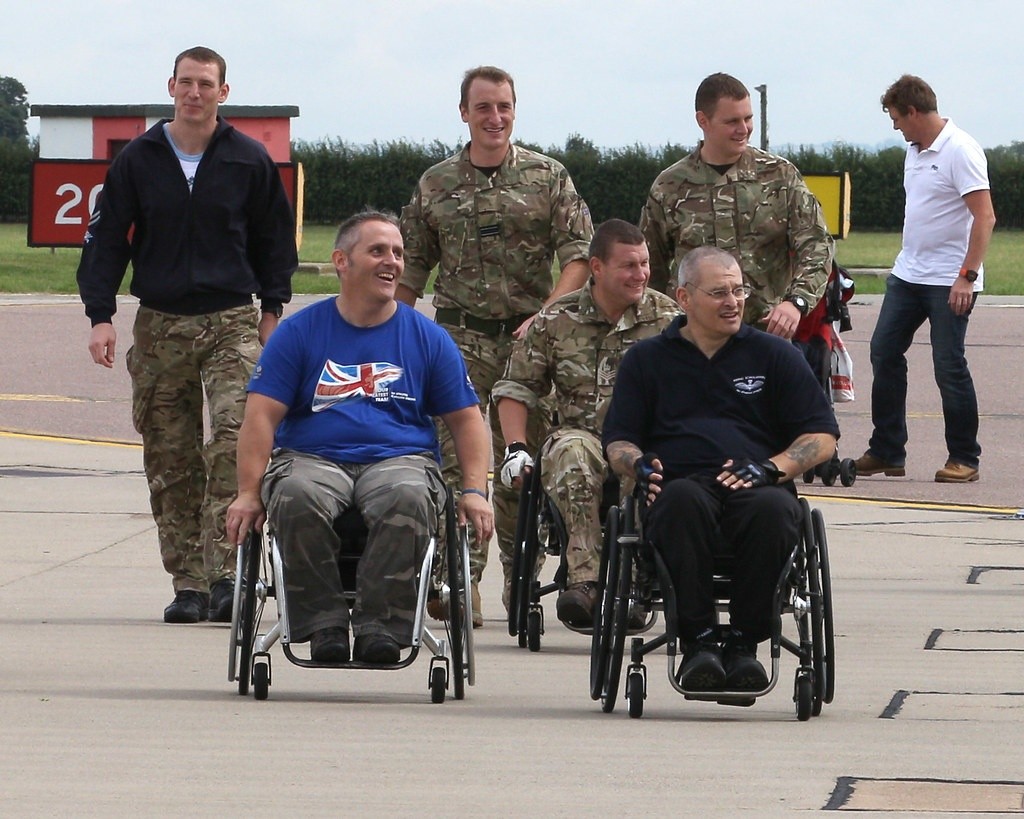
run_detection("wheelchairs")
[502,464,658,652]
[801,259,860,486]
[227,484,479,705]
[588,476,843,721]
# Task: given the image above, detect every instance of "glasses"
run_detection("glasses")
[684,282,751,300]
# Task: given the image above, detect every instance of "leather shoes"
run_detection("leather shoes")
[934,459,980,484]
[852,447,906,477]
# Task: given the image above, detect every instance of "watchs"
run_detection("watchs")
[262,307,283,318]
[959,268,978,282]
[793,296,807,311]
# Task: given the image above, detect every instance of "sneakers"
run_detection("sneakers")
[717,642,769,707]
[206,579,247,624]
[611,583,647,626]
[163,589,210,623]
[310,627,350,662]
[681,645,725,701]
[429,579,484,628]
[352,634,401,665]
[557,582,598,628]
[501,573,511,612]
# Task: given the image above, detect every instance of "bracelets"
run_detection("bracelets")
[462,489,485,497]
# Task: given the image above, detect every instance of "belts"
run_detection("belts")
[434,307,540,336]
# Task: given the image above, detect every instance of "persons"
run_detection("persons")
[76,47,298,622]
[491,219,685,629]
[229,211,495,664]
[638,73,835,344]
[853,75,997,481]
[394,66,596,628]
[601,246,841,708]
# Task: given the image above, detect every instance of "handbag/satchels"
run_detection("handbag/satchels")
[827,322,855,403]
